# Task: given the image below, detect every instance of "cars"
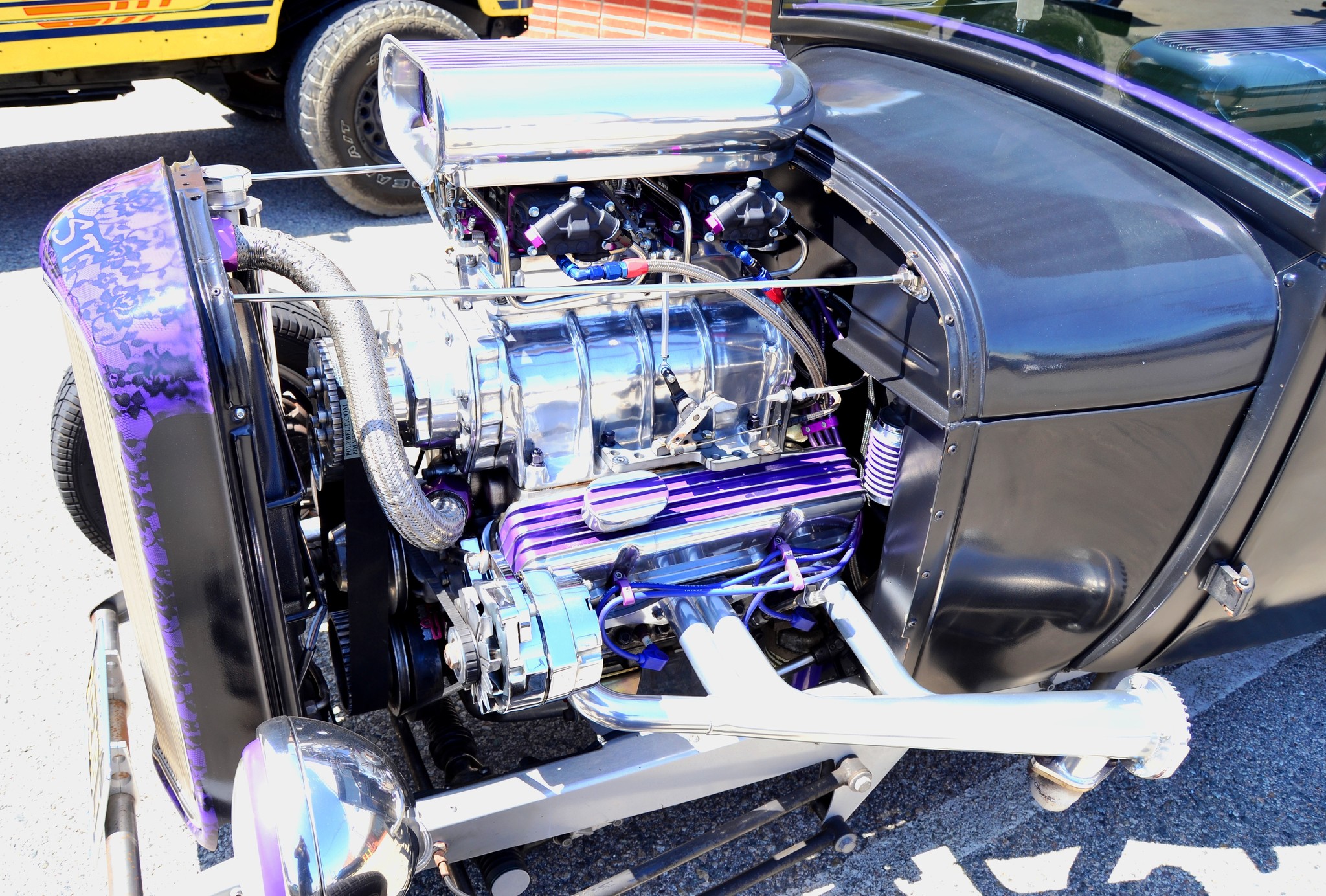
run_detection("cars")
[43,0,1326,896]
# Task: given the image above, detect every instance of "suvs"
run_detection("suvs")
[0,0,532,215]
